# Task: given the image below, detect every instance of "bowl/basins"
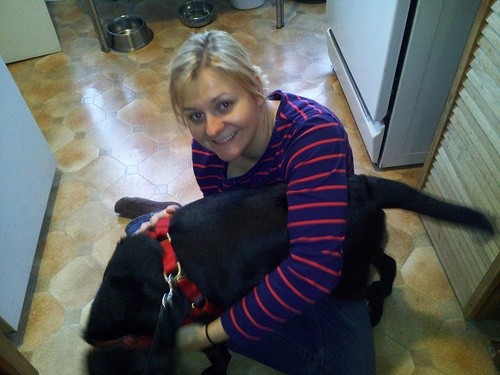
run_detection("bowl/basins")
[177,1,216,28]
[105,13,154,52]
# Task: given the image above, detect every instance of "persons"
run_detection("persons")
[115,30,376,375]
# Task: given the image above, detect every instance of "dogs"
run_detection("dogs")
[80,173,500,375]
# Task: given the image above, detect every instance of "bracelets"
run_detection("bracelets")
[205,323,214,346]
